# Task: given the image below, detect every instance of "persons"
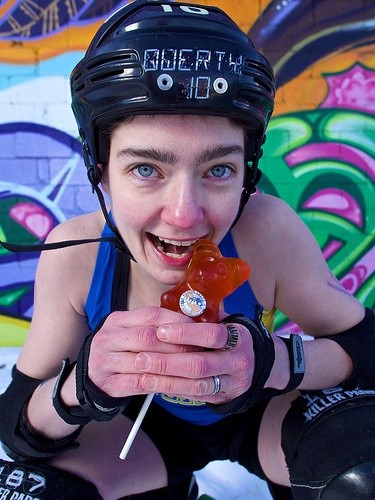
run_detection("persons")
[1,0,375,500]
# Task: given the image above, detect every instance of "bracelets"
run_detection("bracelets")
[51,356,91,426]
[275,333,305,396]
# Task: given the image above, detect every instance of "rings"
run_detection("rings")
[220,322,239,351]
[211,375,221,396]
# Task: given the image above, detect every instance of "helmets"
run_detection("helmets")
[69,0,276,183]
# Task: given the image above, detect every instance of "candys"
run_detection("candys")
[160,238,251,324]
